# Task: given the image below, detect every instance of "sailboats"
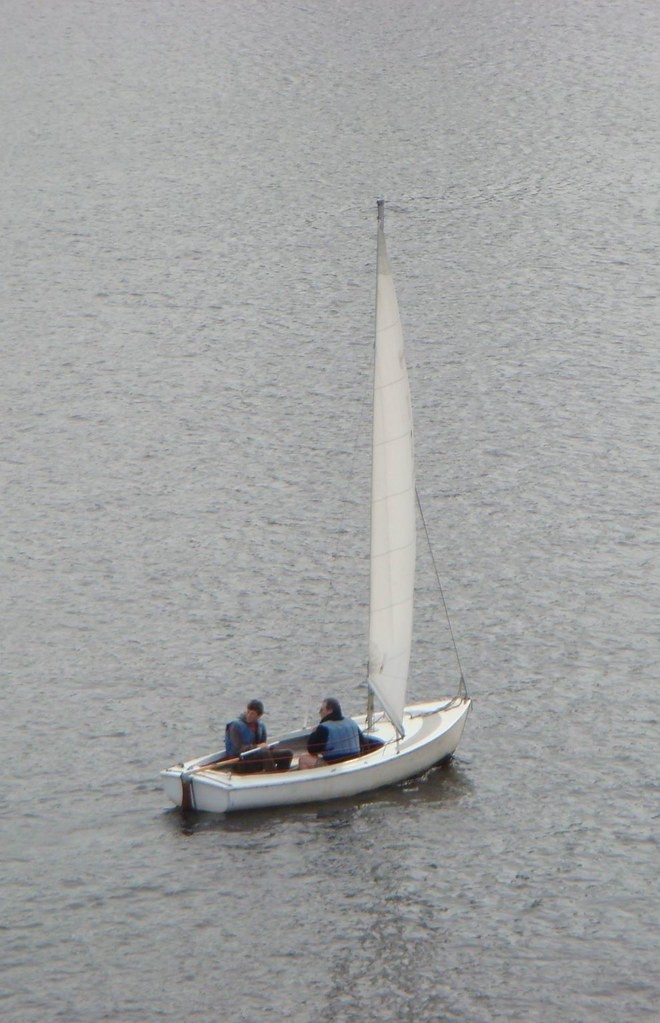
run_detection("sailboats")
[157,200,473,815]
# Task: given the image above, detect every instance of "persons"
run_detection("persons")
[225,699,294,773]
[299,697,369,770]
